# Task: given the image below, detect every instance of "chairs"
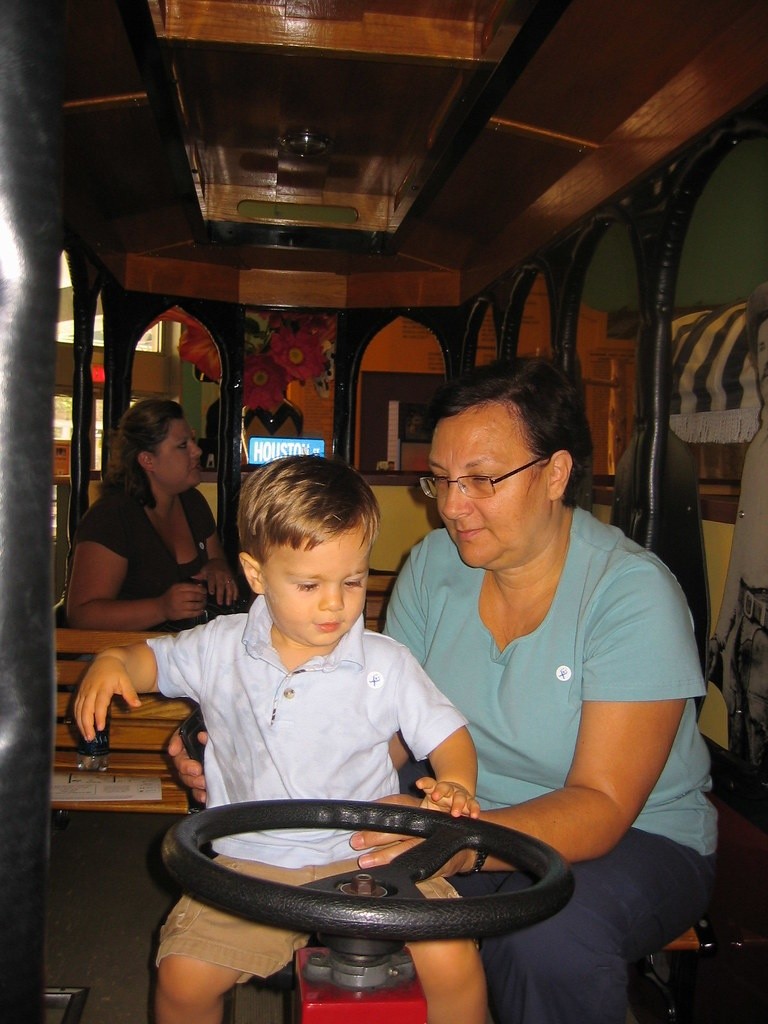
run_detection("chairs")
[47,625,211,829]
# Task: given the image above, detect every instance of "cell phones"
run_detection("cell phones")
[180,707,207,775]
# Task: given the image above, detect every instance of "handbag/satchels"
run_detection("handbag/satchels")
[156,574,251,632]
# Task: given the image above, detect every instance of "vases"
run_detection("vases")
[204,396,303,467]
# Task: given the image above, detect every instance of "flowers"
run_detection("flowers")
[141,304,340,413]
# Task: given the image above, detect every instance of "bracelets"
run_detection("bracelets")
[457,849,489,875]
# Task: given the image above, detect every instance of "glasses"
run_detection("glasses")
[419,457,548,499]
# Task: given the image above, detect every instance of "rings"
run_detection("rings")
[225,580,232,586]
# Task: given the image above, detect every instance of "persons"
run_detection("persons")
[167,353,719,1024]
[65,398,238,633]
[73,454,491,1024]
